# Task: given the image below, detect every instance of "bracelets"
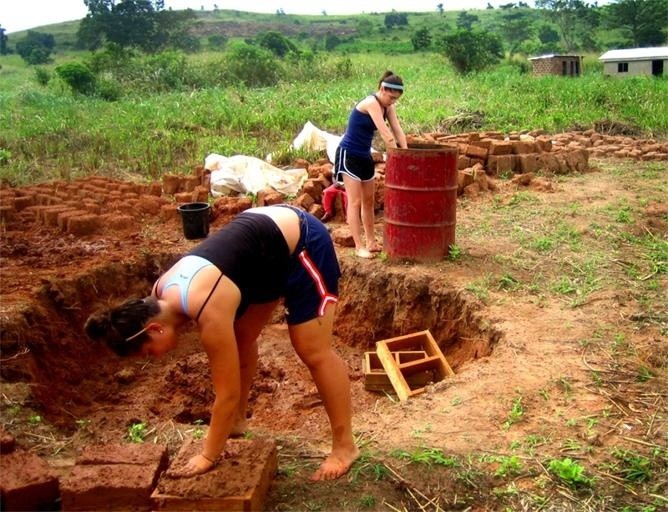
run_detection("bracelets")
[200,452,220,467]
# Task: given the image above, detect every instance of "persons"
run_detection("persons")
[81,203,362,483]
[333,70,408,260]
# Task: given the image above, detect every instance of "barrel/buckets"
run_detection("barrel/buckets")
[176,201,211,241]
[382,141,460,263]
